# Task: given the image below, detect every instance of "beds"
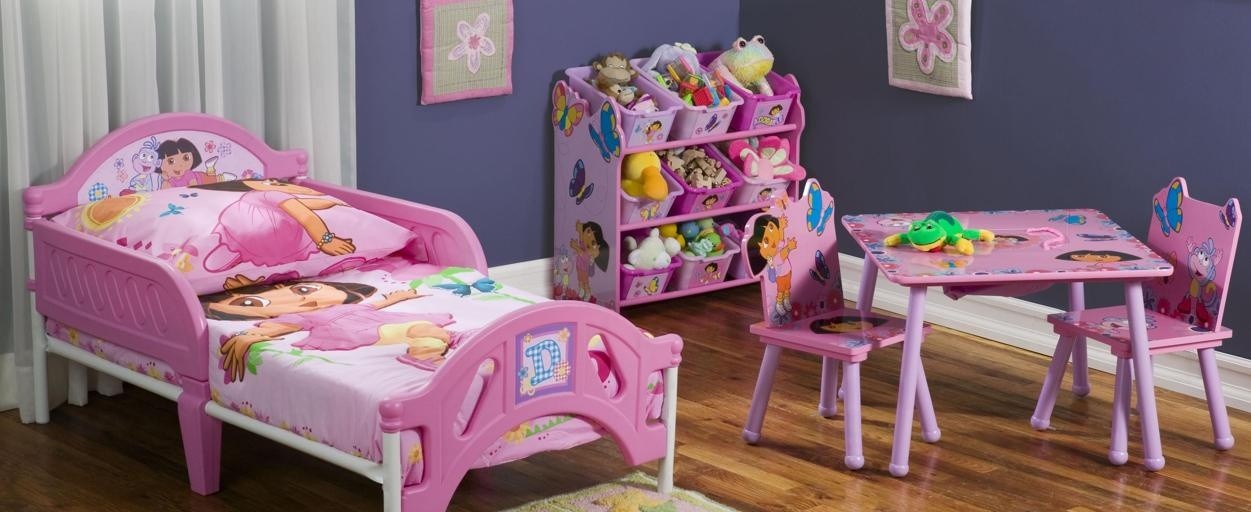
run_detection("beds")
[23,113,684,512]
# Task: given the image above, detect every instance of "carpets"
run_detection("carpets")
[499,471,735,512]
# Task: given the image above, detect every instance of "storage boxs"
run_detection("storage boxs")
[566,63,682,147]
[631,58,742,141]
[670,235,739,294]
[619,168,683,223]
[716,223,750,278]
[697,49,795,131]
[620,229,681,299]
[659,144,741,217]
[711,140,805,205]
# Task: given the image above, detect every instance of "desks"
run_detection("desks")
[841,208,1176,480]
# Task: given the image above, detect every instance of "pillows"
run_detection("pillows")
[50,179,415,296]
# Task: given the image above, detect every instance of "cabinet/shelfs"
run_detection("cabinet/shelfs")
[551,48,805,328]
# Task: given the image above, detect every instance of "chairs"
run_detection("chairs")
[740,180,942,470]
[1032,176,1243,465]
